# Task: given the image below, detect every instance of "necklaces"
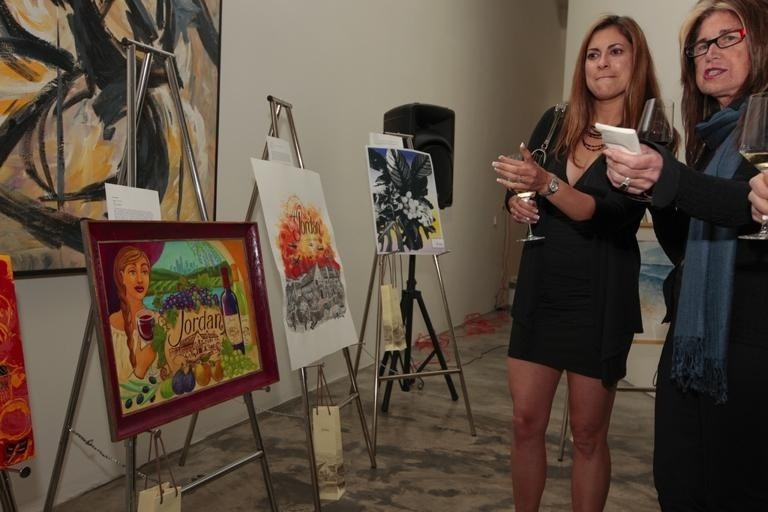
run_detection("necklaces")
[582,122,622,151]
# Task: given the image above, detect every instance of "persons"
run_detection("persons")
[746,171,768,226]
[601,3,768,511]
[490,12,676,509]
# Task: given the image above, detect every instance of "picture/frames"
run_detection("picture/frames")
[632,222,676,344]
[79,218,280,442]
[0,0,222,282]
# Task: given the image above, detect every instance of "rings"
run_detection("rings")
[617,177,630,192]
[526,217,530,221]
[760,215,768,226]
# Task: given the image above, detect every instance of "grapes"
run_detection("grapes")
[124,368,168,409]
[159,286,220,316]
[220,349,258,379]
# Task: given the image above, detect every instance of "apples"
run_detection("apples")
[191,358,211,386]
[209,359,224,381]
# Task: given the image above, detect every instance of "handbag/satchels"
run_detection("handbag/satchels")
[380,254,407,352]
[310,363,348,502]
[137,427,182,512]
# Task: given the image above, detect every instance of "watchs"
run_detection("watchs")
[537,171,559,197]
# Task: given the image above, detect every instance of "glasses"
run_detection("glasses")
[682,26,745,60]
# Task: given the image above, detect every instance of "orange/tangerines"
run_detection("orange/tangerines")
[160,378,175,398]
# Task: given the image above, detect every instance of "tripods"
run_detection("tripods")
[378,255,459,412]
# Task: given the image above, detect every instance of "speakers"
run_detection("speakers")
[383,102,455,209]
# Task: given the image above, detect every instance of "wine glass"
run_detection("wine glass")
[629,99,675,202]
[738,92,768,240]
[507,154,546,243]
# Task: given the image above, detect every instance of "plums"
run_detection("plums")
[172,370,195,394]
